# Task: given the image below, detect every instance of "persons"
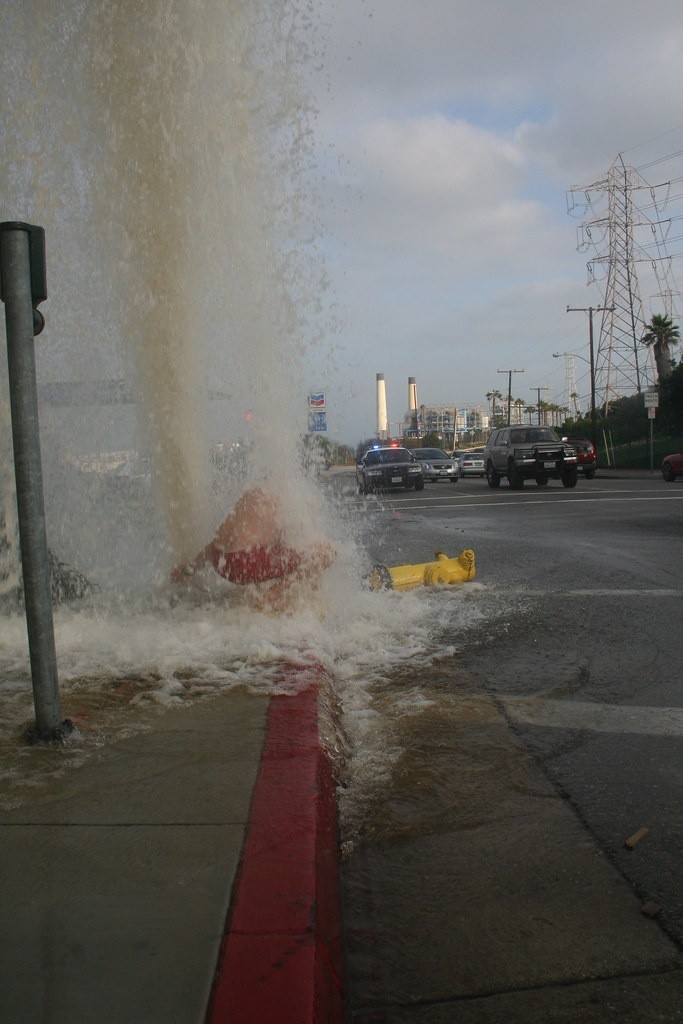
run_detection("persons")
[169,445,338,615]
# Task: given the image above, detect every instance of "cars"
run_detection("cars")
[660,450,683,483]
[449,451,473,465]
[355,444,424,495]
[457,452,486,479]
[408,447,461,483]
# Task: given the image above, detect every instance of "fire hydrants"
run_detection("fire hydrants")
[359,549,476,594]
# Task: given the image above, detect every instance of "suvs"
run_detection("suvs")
[563,436,596,480]
[483,425,579,491]
